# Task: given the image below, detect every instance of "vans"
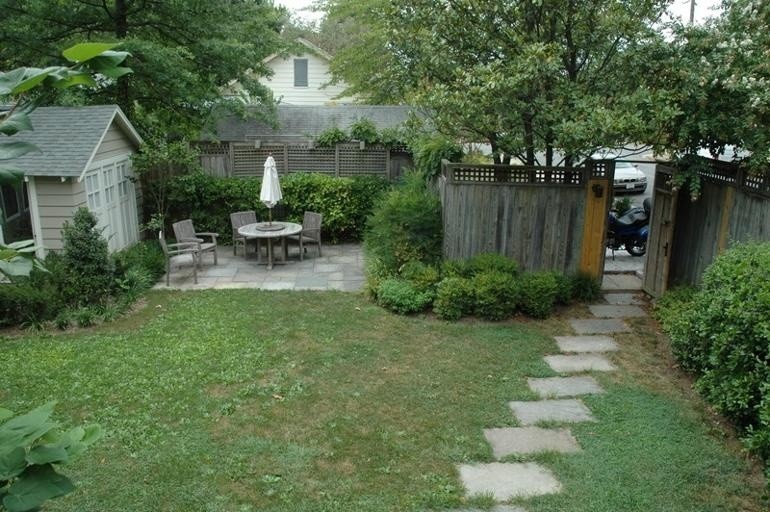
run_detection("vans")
[701,137,754,165]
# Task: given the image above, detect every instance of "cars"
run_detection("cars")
[575,152,647,196]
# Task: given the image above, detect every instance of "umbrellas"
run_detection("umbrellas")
[255,153,287,231]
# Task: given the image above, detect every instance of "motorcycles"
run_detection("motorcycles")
[605,197,652,261]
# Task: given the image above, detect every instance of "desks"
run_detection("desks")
[237,221,302,271]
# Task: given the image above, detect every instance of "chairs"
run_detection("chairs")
[230,210,260,259]
[172,219,219,269]
[159,230,200,287]
[286,210,322,261]
